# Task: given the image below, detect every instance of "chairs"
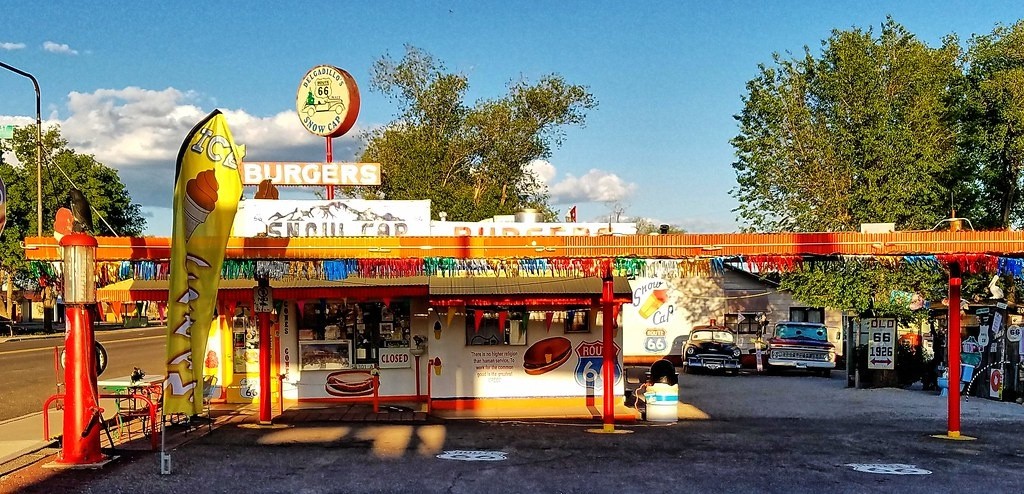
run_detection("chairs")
[169,375,218,438]
[115,381,163,447]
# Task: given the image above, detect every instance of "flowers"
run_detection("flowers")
[413,335,428,350]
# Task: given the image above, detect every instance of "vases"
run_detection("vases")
[410,349,424,356]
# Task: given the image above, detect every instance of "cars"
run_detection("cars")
[766,321,837,374]
[680,318,743,377]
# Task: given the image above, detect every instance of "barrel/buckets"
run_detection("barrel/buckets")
[646,383,678,422]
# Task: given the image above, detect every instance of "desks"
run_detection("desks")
[97,375,166,432]
